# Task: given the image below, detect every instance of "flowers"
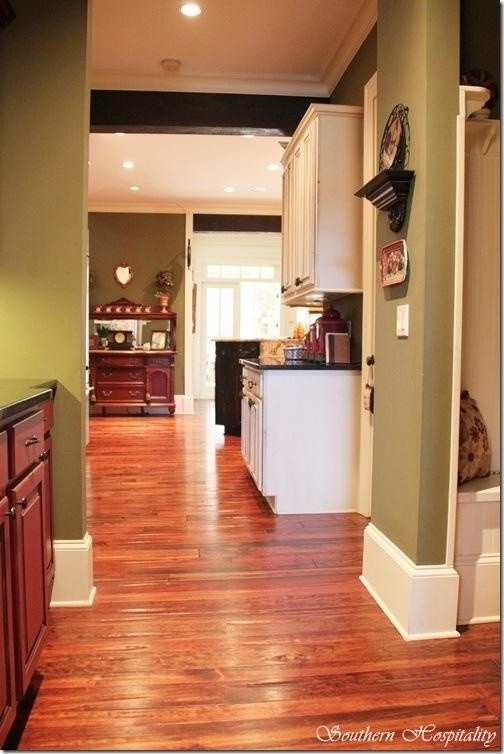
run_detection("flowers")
[154,271,178,298]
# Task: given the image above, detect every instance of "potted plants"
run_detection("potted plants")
[97,326,109,347]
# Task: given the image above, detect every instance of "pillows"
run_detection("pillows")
[458,390,491,486]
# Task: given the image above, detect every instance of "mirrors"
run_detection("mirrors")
[112,262,138,288]
[89,318,171,350]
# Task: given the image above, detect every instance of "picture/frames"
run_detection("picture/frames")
[150,330,168,351]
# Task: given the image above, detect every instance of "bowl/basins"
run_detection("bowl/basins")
[283,348,307,361]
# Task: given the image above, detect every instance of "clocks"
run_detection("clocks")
[109,330,134,349]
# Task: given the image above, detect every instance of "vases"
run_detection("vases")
[159,291,170,313]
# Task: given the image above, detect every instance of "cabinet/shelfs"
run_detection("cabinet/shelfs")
[278,103,364,307]
[88,348,177,418]
[241,364,362,515]
[0,395,54,750]
